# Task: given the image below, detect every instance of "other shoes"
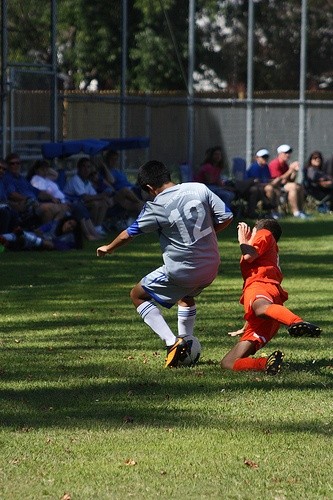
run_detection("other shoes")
[0,234,8,245]
[271,212,280,220]
[14,227,24,238]
[294,210,310,218]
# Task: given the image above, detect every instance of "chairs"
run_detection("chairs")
[179,158,333,216]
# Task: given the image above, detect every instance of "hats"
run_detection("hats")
[256,149,270,157]
[277,144,291,153]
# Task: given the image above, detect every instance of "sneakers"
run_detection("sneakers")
[166,337,187,367]
[264,350,285,375]
[289,322,322,337]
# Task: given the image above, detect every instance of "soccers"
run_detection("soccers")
[176,334,201,366]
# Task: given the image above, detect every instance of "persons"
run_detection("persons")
[96,160,235,367]
[222,217,322,374]
[0,145,333,249]
